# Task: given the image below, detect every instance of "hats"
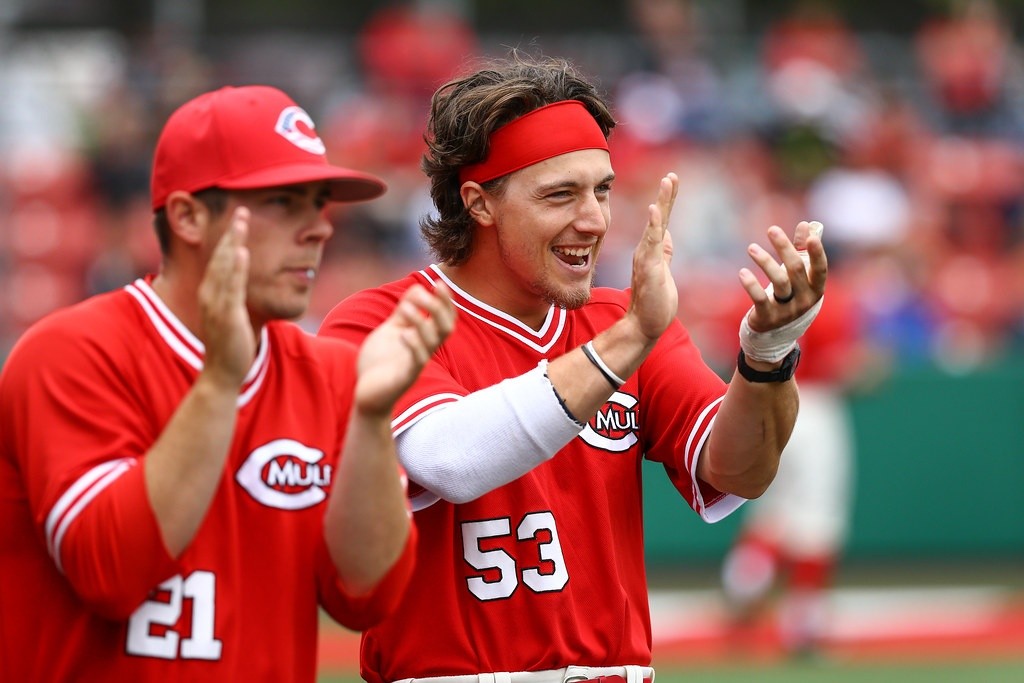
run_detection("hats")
[151,85,387,212]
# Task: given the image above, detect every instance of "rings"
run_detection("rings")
[774,287,794,303]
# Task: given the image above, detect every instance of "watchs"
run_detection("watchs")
[738,342,801,383]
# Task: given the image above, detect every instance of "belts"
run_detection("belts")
[391,665,654,683]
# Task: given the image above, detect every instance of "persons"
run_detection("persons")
[0,87,457,683]
[0,0,1024,683]
[316,46,827,683]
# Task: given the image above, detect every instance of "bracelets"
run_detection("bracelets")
[580,345,620,390]
[587,340,626,385]
